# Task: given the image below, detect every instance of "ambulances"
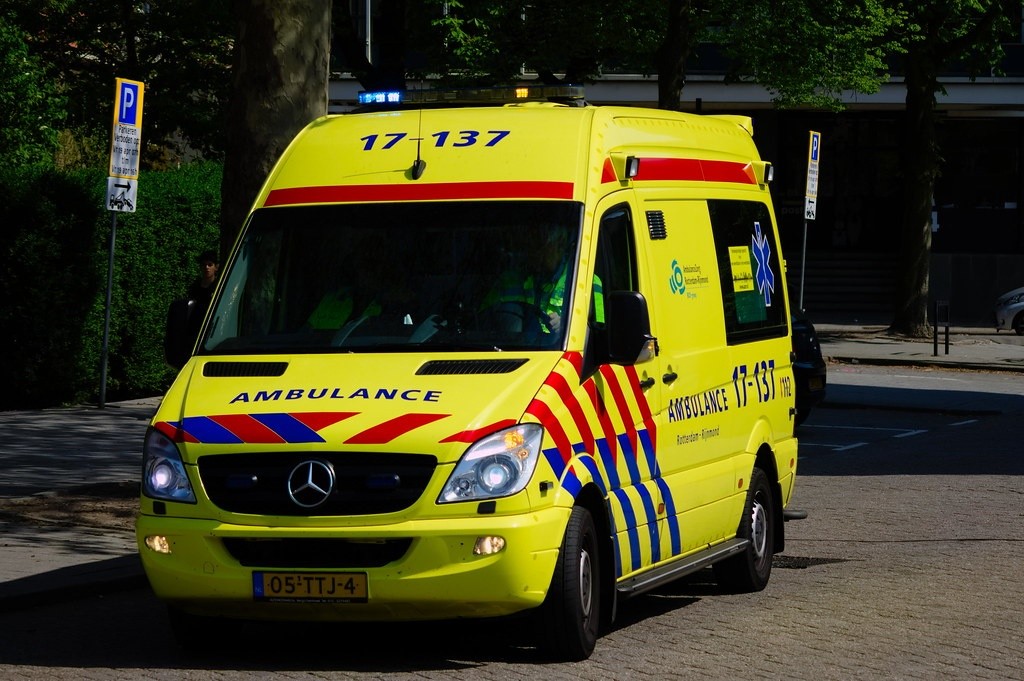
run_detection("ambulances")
[135,85,810,663]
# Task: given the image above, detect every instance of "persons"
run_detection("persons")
[186,251,218,304]
[477,217,608,333]
[301,249,381,331]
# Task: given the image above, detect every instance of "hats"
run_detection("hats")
[193,251,222,266]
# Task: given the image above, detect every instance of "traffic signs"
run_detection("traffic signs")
[802,193,818,220]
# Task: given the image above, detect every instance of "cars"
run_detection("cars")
[781,301,827,428]
[994,284,1024,340]
